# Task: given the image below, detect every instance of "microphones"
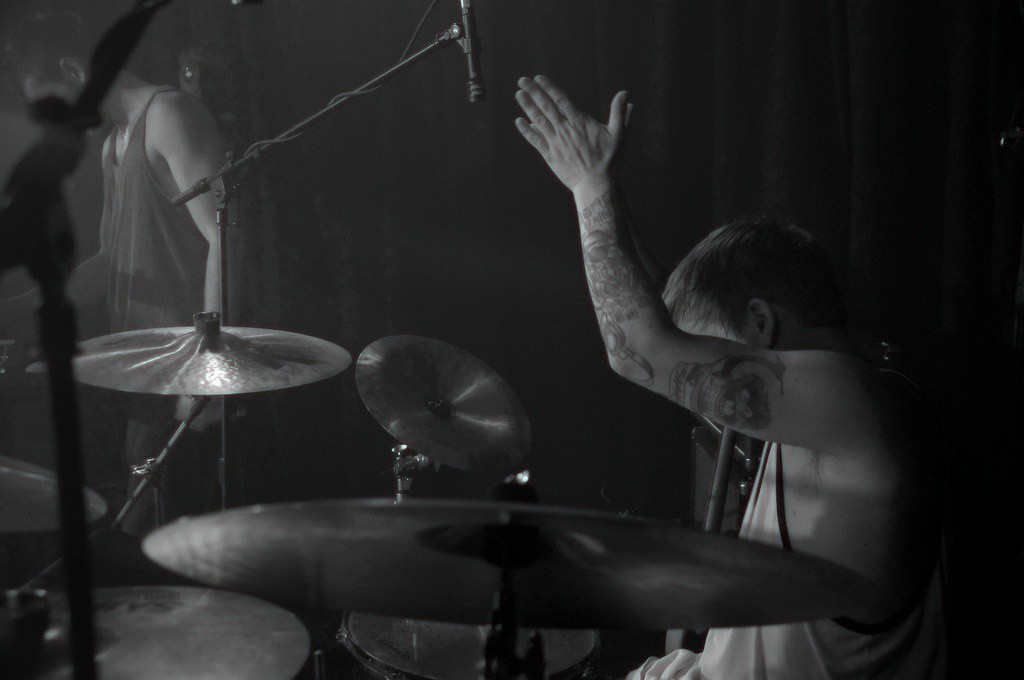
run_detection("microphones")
[460,0,486,103]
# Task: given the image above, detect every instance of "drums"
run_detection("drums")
[337,611,605,678]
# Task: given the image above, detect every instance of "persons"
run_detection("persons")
[15,9,243,539]
[515,76,945,680]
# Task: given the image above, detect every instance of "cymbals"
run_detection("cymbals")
[0,455,109,534]
[141,498,879,629]
[0,582,311,679]
[355,334,534,476]
[74,325,352,397]
